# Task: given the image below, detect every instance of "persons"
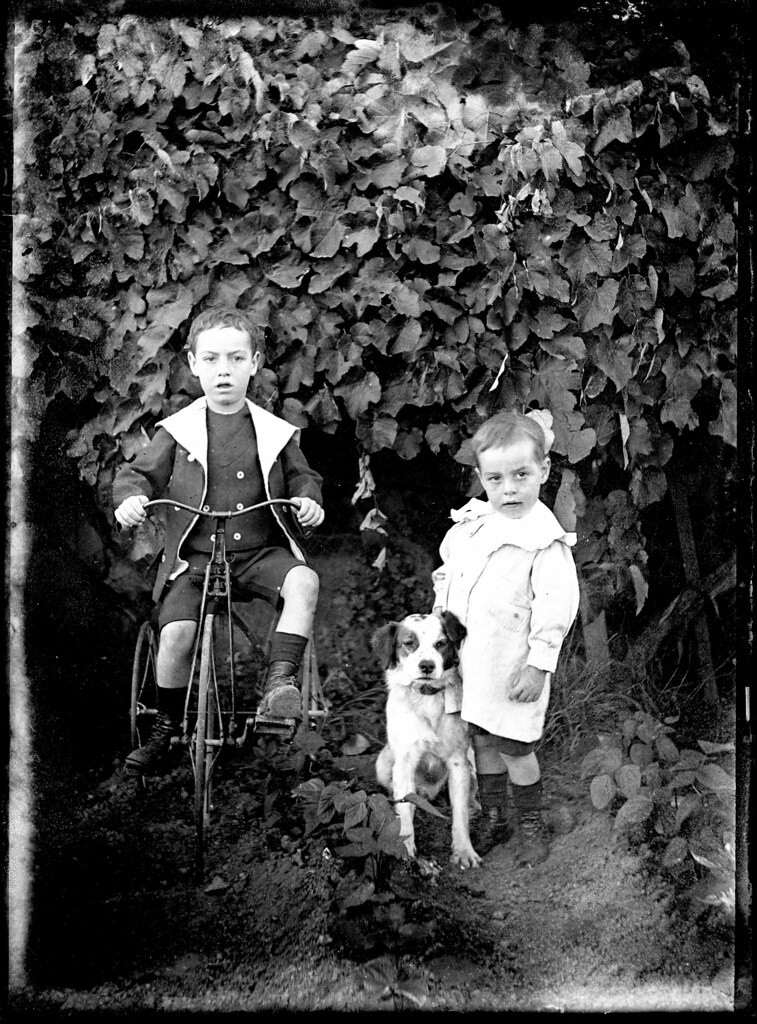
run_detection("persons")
[430,414,580,868]
[110,308,324,776]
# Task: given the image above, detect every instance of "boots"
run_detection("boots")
[125,714,180,769]
[518,809,548,868]
[468,800,512,856]
[255,661,302,763]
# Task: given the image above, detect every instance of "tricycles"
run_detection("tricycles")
[130,498,331,884]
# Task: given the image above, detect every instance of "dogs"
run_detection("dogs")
[369,607,486,873]
